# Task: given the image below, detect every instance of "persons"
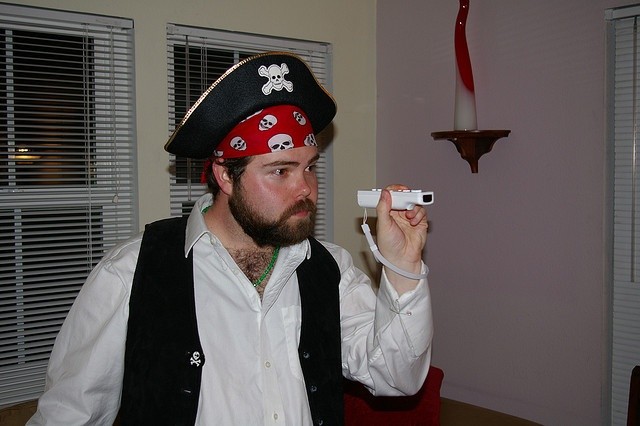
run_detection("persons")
[25,52,434,426]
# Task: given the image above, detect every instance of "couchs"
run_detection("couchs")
[342,365,445,426]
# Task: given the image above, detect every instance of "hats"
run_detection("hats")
[164,51,337,159]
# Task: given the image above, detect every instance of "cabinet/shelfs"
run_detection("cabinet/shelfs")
[430,128,511,174]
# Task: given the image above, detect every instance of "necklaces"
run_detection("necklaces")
[222,243,279,288]
